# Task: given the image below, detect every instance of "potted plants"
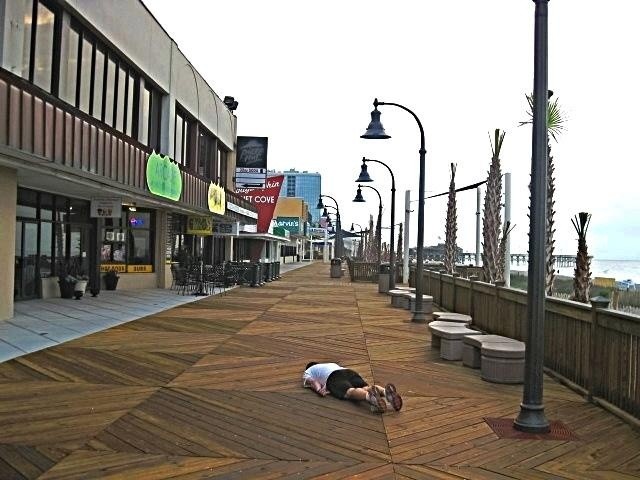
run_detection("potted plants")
[102,269,121,291]
[57,256,90,299]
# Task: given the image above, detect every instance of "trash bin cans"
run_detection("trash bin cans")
[265,262,282,282]
[330,258,342,279]
[378,262,396,294]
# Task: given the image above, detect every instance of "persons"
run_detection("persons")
[302,362,404,414]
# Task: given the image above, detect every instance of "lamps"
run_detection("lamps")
[128,203,138,212]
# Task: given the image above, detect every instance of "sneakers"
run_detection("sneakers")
[385,383,403,412]
[367,385,387,413]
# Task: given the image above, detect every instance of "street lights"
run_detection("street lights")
[353,184,382,284]
[360,98,429,322]
[355,157,393,291]
[316,194,346,277]
[350,223,363,259]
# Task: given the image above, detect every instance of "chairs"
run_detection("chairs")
[168,257,249,298]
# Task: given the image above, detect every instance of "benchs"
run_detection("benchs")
[388,282,432,313]
[428,310,525,385]
